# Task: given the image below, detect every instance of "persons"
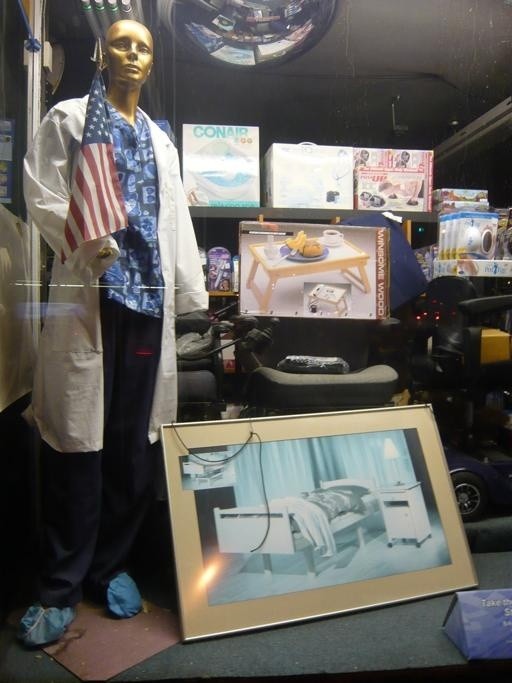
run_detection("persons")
[20,19,211,648]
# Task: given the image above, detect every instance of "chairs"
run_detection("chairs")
[234,319,402,407]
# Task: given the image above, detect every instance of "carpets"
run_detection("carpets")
[9,578,182,682]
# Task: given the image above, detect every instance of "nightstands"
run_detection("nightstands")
[379,483,432,548]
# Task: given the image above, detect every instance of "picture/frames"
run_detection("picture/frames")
[157,402,480,640]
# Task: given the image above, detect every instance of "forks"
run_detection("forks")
[272,248,297,268]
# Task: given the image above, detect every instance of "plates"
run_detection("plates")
[281,242,329,261]
[320,237,342,247]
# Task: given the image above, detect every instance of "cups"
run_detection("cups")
[323,230,344,242]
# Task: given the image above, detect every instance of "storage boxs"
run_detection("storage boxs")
[262,141,353,212]
[353,148,435,212]
[238,221,390,322]
[182,122,261,208]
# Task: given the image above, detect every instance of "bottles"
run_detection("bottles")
[231,254,239,293]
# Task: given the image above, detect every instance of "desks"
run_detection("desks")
[245,238,370,312]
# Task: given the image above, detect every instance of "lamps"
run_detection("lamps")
[383,437,405,487]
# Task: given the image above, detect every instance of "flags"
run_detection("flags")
[57,73,129,265]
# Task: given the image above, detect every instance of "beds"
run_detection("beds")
[213,478,377,577]
[182,457,228,483]
